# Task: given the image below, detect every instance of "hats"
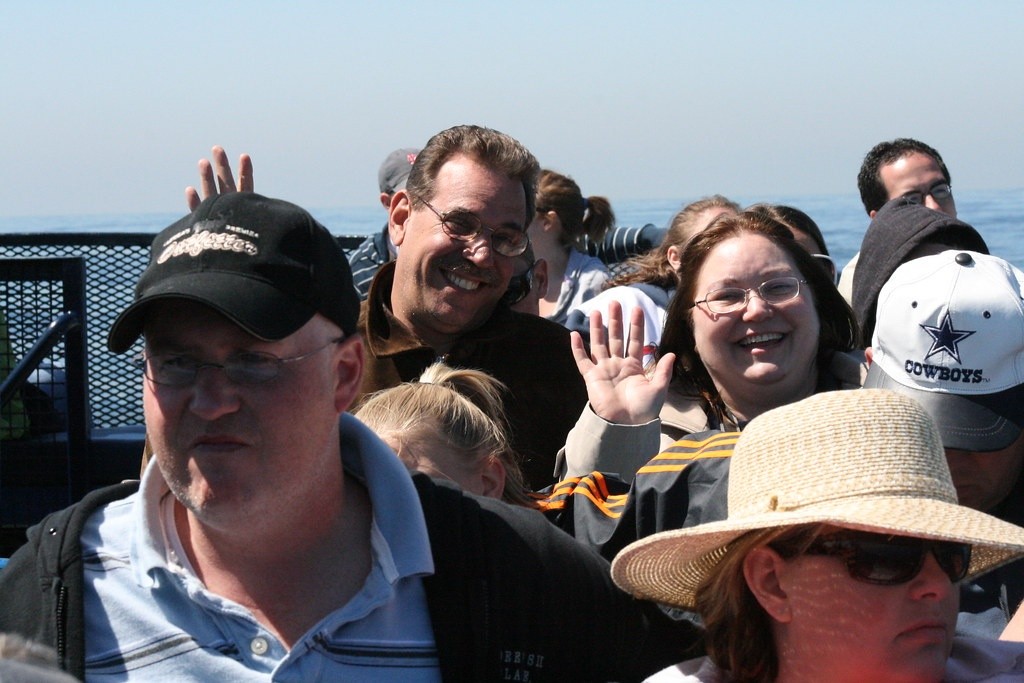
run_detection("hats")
[862,251,1024,452]
[108,191,360,361]
[608,390,1024,615]
[378,149,424,197]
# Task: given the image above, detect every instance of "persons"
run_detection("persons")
[347,359,741,564]
[863,250,1024,642]
[608,389,1024,683]
[184,124,991,485]
[0,191,711,683]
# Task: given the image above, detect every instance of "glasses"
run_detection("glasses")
[138,336,352,388]
[411,195,530,258]
[768,527,974,585]
[688,277,807,315]
[900,184,953,207]
